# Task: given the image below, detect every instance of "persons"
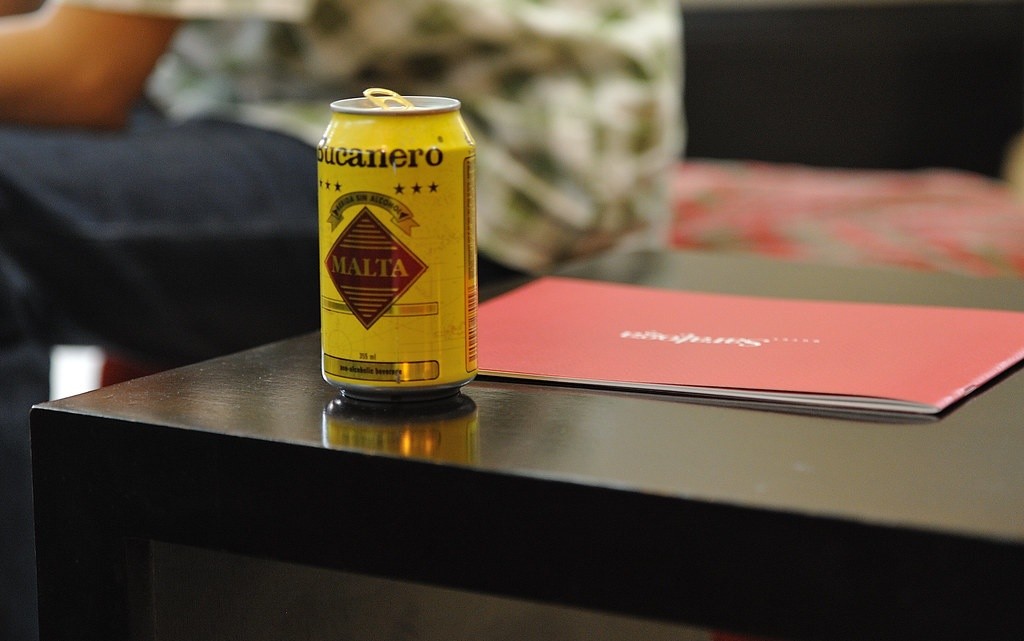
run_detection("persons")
[0,1,690,641]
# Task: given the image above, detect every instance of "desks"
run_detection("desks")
[30,242,1024,641]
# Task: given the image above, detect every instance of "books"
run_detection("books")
[476,273,1023,415]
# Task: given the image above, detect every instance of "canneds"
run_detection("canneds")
[316,88,479,402]
